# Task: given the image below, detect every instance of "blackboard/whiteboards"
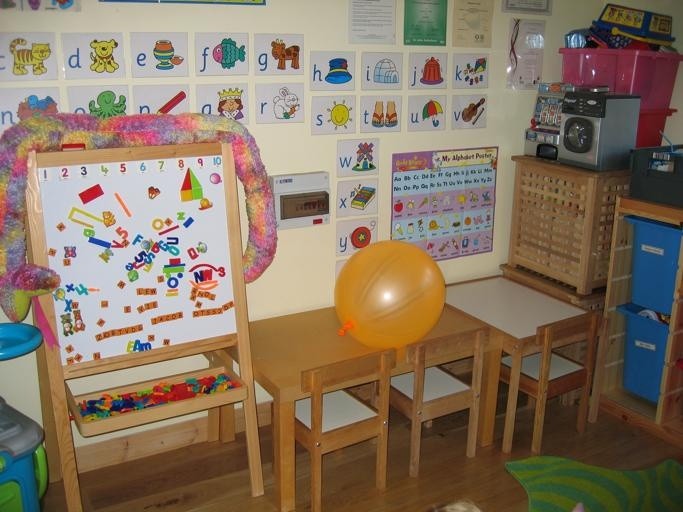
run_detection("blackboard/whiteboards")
[24,142,247,380]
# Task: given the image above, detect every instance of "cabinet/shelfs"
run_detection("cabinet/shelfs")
[498,152,630,410]
[589,192,681,450]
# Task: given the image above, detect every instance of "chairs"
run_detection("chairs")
[378,327,493,478]
[268,344,400,511]
[499,304,605,455]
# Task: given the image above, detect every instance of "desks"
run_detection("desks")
[217,302,504,510]
[440,271,591,459]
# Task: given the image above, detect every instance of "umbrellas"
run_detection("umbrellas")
[420,98,443,127]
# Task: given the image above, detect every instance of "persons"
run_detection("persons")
[430,499,481,512]
[372,101,398,128]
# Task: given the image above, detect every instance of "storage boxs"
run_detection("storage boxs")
[624,217,683,311]
[556,46,682,112]
[615,302,669,404]
[627,145,680,206]
[634,107,677,149]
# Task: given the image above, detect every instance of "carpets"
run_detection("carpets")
[502,454,682,511]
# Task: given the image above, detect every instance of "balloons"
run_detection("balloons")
[333,240,446,350]
